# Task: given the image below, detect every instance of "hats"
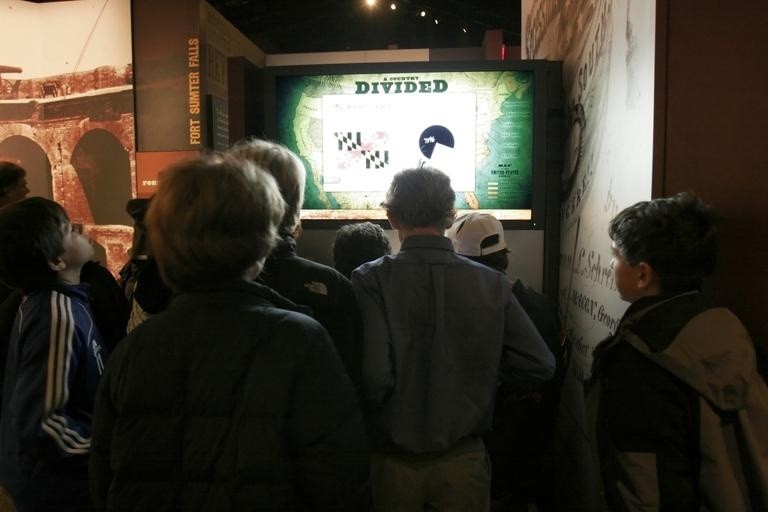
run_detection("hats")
[448,212,506,256]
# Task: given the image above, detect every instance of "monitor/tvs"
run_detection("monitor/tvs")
[260,63,550,232]
